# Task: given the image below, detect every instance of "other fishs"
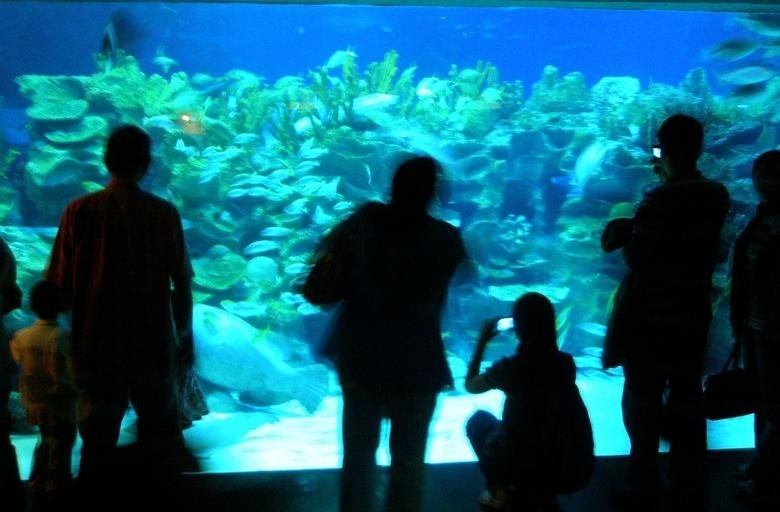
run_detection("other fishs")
[1,11,780,382]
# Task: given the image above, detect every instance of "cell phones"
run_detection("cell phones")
[652,146,661,159]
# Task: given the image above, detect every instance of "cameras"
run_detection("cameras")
[497,317,515,331]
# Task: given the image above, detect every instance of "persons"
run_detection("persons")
[729,145,779,510]
[8,275,81,502]
[598,108,734,511]
[299,151,468,512]
[0,235,27,512]
[41,121,198,509]
[462,290,596,511]
